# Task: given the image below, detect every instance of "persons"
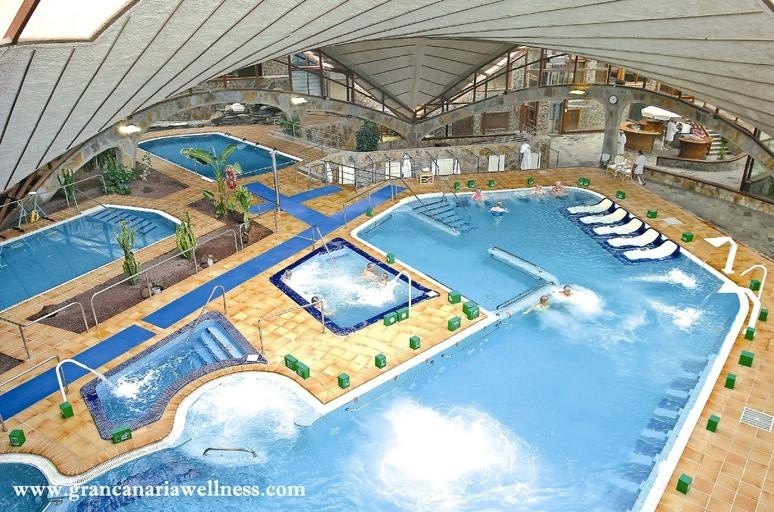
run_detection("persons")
[381,272,389,286]
[471,188,484,201]
[533,185,544,194]
[551,181,564,193]
[520,141,531,161]
[491,201,504,212]
[311,295,334,315]
[524,295,549,313]
[635,150,646,185]
[559,285,573,297]
[617,131,627,155]
[665,118,706,148]
[282,269,292,282]
[362,262,376,279]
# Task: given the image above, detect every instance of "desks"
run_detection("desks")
[619,117,713,160]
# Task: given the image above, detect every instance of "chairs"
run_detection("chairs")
[566,197,680,263]
[605,154,635,185]
